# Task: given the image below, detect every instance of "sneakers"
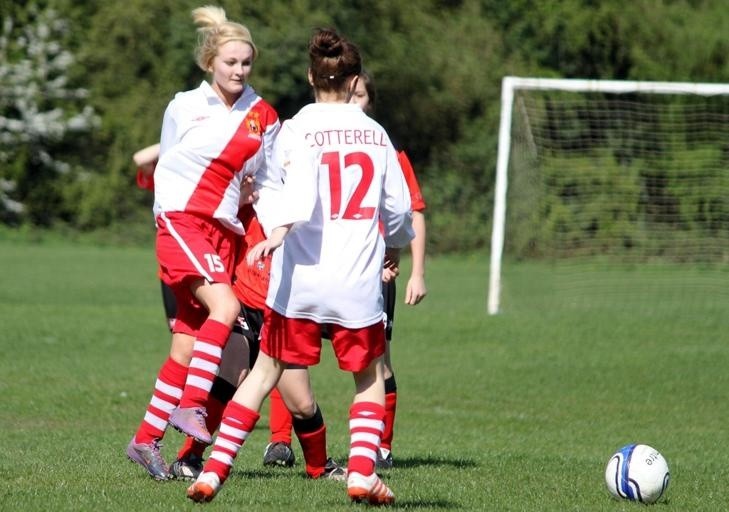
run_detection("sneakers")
[320,457,348,480]
[377,448,393,466]
[166,453,203,481]
[168,403,213,445]
[264,441,296,466]
[187,471,221,503]
[125,436,174,482]
[347,471,395,504]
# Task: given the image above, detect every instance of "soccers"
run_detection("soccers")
[605,444,669,504]
